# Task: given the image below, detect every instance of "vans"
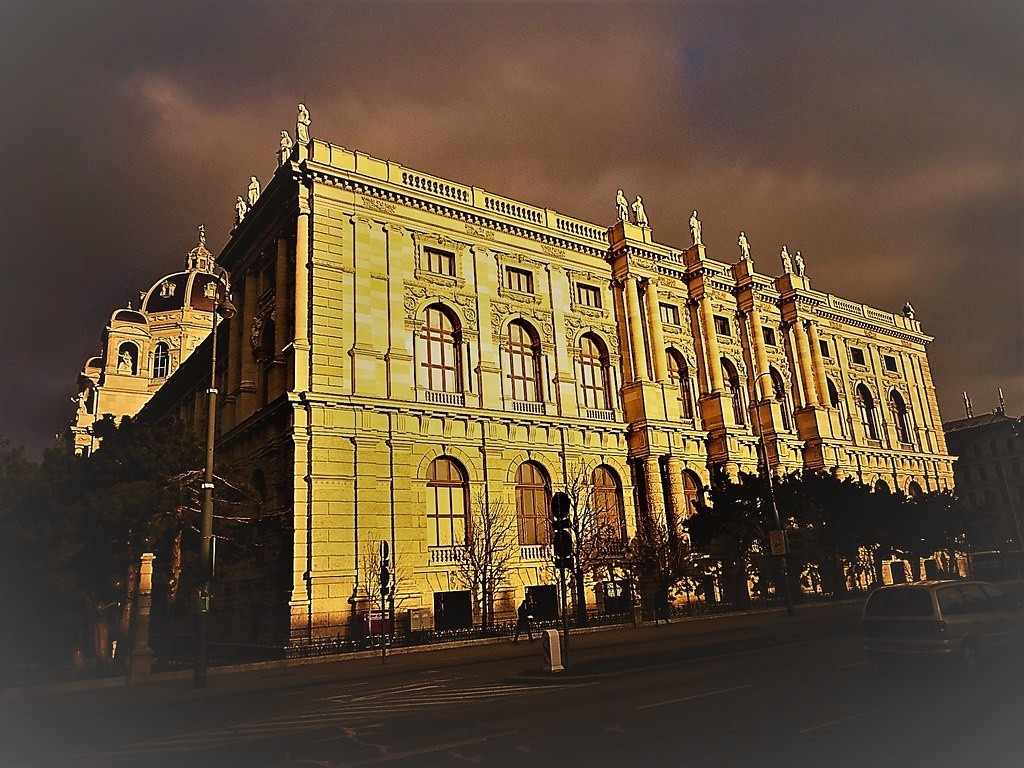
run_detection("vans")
[862,578,1023,671]
[970,550,1022,573]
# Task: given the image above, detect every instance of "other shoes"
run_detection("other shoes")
[529,639,536,644]
[514,641,520,645]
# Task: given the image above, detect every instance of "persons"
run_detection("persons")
[513,600,537,645]
[654,590,672,626]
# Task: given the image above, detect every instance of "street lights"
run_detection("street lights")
[988,415,1023,577]
[752,373,796,617]
[192,269,238,690]
